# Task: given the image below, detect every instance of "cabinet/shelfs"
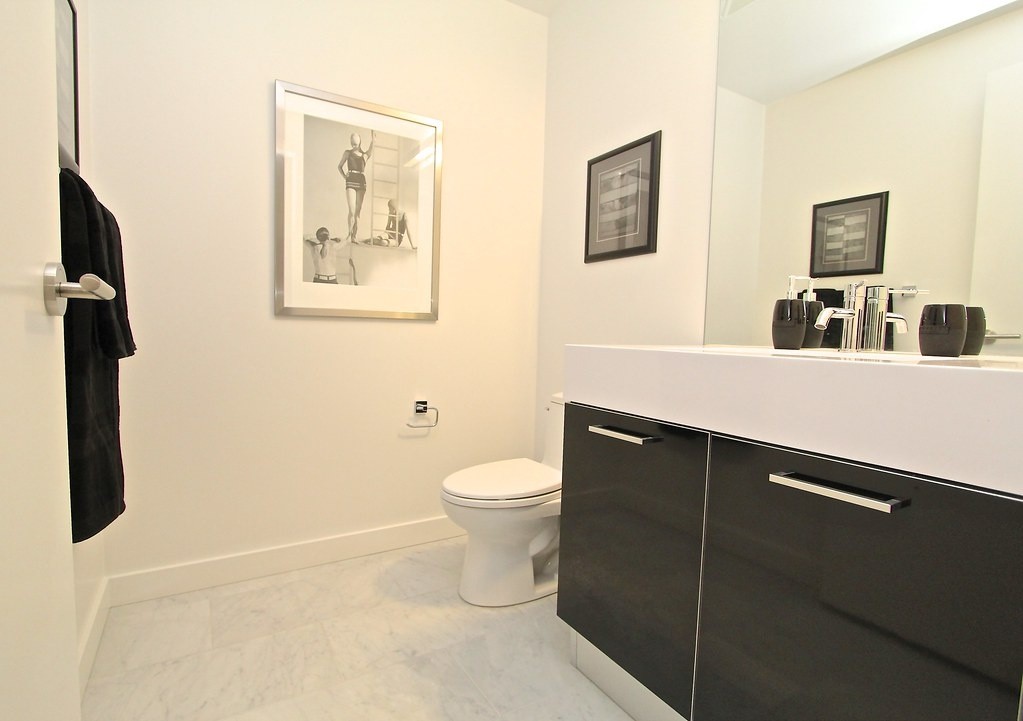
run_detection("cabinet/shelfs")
[551,401,1023,721]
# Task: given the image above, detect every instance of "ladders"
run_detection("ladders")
[370,130,400,248]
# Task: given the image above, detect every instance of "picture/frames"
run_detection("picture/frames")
[583,130,661,263]
[810,192,888,275]
[54,0,80,174]
[275,81,438,322]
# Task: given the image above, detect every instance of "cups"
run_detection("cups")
[772,299,806,349]
[801,301,825,348]
[919,304,967,357]
[962,307,986,355]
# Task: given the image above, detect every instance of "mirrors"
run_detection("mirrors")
[701,0,1022,356]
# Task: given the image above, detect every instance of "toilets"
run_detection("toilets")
[440,391,565,607]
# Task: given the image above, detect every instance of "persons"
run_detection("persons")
[338,129,376,244]
[386,200,417,249]
[303,227,348,284]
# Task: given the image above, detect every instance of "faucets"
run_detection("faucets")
[813,278,867,354]
[863,284,918,351]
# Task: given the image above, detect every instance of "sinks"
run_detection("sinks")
[700,346,910,362]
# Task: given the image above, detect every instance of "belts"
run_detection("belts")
[348,169,364,174]
[315,276,338,281]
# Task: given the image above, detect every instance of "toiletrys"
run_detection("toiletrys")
[794,277,826,349]
[771,275,811,349]
